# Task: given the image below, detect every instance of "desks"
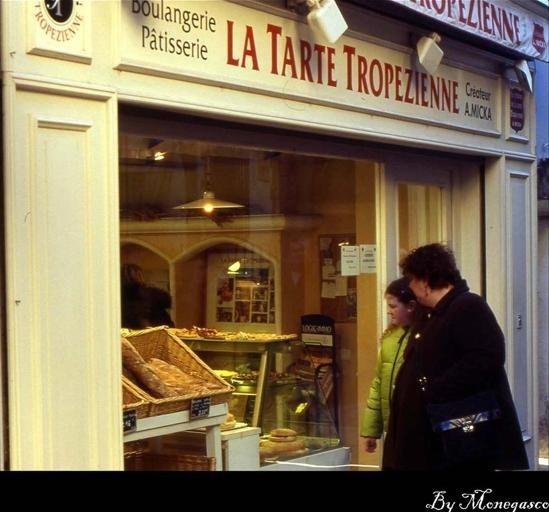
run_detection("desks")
[122,401,229,474]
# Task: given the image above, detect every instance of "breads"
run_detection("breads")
[260,429,309,457]
[122,337,221,399]
[221,412,235,431]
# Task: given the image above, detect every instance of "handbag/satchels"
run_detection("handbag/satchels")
[430,388,504,456]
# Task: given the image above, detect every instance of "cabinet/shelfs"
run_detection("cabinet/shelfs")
[163,425,263,473]
[162,327,351,471]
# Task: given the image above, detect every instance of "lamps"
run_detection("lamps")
[414,31,445,75]
[171,143,245,216]
[498,58,533,93]
[306,0,349,46]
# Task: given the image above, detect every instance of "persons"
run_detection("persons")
[381,242,530,472]
[359,276,424,453]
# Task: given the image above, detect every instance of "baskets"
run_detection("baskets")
[123,325,236,419]
